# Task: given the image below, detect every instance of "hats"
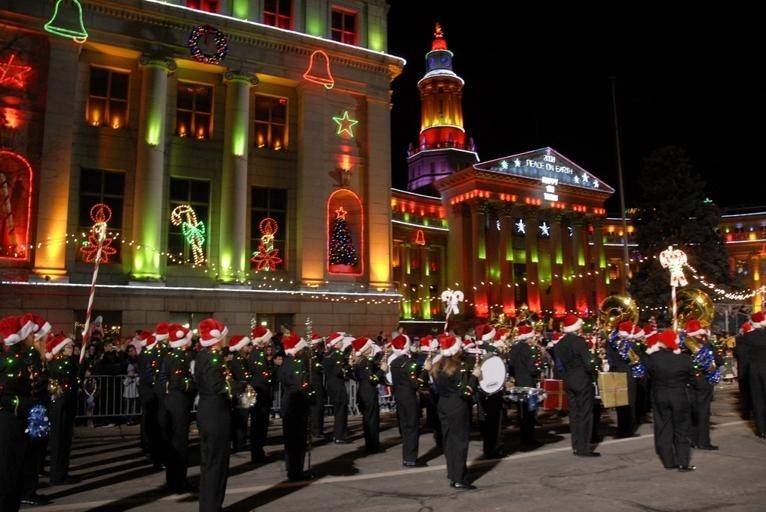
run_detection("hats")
[326,331,345,347]
[563,310,583,332]
[252,326,273,344]
[391,334,410,355]
[0,311,73,359]
[229,335,250,351]
[437,334,462,357]
[138,322,192,350]
[351,336,373,356]
[282,333,306,356]
[310,330,323,344]
[517,325,535,341]
[198,318,230,347]
[419,334,437,351]
[616,310,766,355]
[474,325,497,345]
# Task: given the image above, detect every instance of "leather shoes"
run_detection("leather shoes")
[679,463,697,473]
[692,443,719,451]
[403,461,428,469]
[285,474,315,481]
[333,437,352,446]
[572,449,601,457]
[20,492,47,506]
[450,480,477,492]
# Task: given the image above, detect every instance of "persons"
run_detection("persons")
[1,312,766,512]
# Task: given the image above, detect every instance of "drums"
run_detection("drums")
[374,349,397,387]
[415,351,440,394]
[459,348,508,395]
[349,343,381,367]
[339,337,354,354]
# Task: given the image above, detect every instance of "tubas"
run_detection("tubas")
[599,296,639,363]
[668,289,716,374]
